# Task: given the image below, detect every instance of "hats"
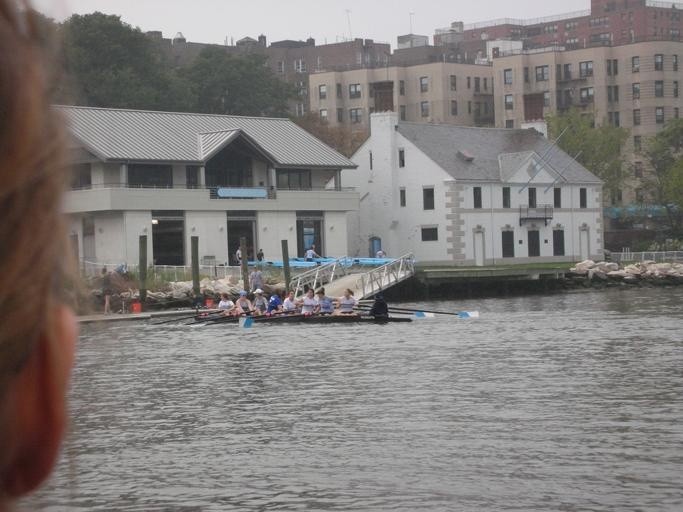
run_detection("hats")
[315,287,325,295]
[239,290,247,297]
[252,289,264,295]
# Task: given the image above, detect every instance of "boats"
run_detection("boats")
[193,311,360,324]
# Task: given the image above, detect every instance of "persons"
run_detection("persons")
[311,244,320,258]
[255,249,264,261]
[248,265,265,292]
[0,0,83,510]
[234,246,244,266]
[99,267,113,317]
[375,250,386,258]
[216,286,361,317]
[303,246,322,262]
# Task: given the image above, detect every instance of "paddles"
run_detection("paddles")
[238,312,332,327]
[334,307,434,317]
[354,305,479,317]
